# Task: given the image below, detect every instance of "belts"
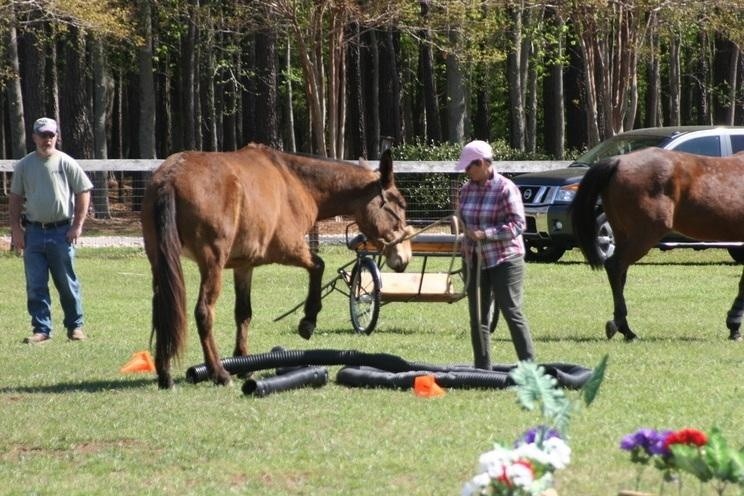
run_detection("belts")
[21,218,70,230]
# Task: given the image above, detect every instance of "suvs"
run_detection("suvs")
[507,123,743,266]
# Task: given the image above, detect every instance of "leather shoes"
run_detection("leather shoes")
[24,326,84,344]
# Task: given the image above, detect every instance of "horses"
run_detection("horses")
[570,147,744,343]
[140,141,413,392]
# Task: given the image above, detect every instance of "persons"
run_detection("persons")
[9,117,94,343]
[453,141,535,371]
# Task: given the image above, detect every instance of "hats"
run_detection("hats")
[34,118,57,135]
[454,139,494,170]
